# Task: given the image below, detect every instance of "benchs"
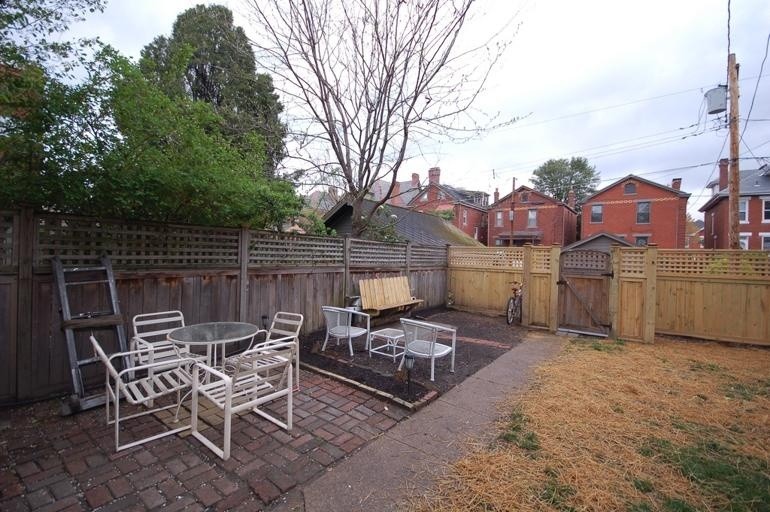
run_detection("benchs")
[358,275,424,328]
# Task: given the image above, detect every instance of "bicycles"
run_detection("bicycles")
[506,280,522,325]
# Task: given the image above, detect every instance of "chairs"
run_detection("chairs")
[90,309,304,462]
[321,301,457,383]
